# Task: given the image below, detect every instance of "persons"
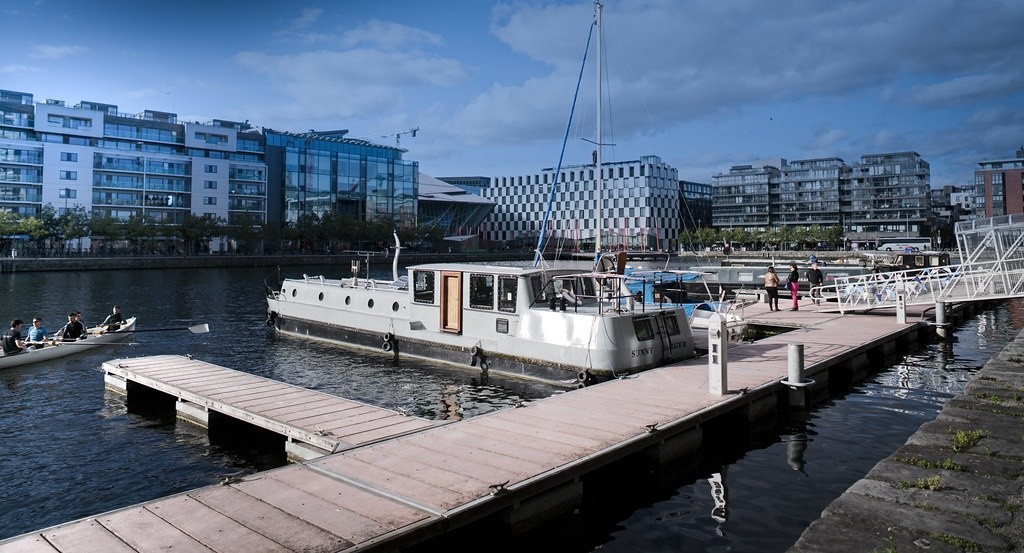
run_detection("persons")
[100,304,127,332]
[54,311,88,342]
[24,317,48,350]
[763,266,782,312]
[788,263,799,311]
[806,262,823,306]
[2,319,27,356]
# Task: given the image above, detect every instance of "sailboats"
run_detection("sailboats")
[267,1,698,388]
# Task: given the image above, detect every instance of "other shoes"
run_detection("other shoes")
[770,307,773,311]
[776,308,781,311]
[813,300,820,306]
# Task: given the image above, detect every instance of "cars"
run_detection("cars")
[871,252,958,284]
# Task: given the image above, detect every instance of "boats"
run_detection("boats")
[1,311,142,369]
[669,297,739,343]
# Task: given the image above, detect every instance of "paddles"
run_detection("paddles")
[88,322,214,335]
[22,338,142,348]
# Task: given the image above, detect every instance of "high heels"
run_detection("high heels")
[789,307,798,311]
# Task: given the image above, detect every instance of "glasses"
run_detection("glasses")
[33,321,39,323]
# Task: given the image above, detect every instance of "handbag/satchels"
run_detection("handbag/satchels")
[784,282,790,290]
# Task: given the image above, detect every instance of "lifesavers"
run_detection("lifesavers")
[381,331,392,352]
[468,346,479,367]
[266,310,276,326]
